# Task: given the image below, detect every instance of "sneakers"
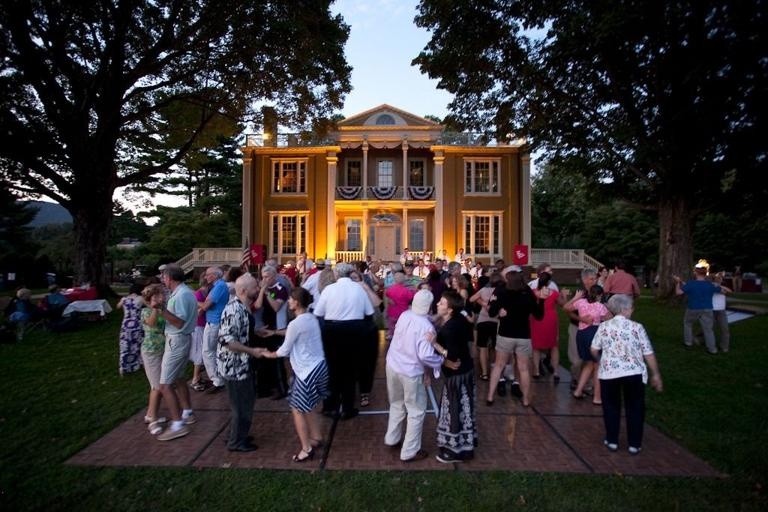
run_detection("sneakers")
[182,412,197,424]
[685,339,694,346]
[498,378,522,397]
[707,345,728,355]
[157,423,190,441]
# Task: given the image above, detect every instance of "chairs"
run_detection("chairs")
[721,272,762,292]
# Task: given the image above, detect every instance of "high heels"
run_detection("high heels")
[293,449,315,464]
[312,436,325,449]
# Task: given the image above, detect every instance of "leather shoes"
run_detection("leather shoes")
[224,434,258,452]
[322,407,359,421]
[436,451,464,463]
[207,384,225,393]
[539,358,554,375]
[400,449,428,464]
[570,379,593,391]
[384,436,402,447]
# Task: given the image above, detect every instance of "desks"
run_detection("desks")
[62,299,113,328]
[29,292,66,310]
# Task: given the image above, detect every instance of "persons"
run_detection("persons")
[117,284,149,376]
[731,265,743,291]
[673,264,728,355]
[48,284,80,324]
[15,287,47,322]
[707,274,733,353]
[4,286,24,315]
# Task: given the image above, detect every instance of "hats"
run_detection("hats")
[412,289,434,316]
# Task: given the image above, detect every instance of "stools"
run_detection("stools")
[24,317,46,337]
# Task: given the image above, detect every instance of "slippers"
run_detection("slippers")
[532,368,542,378]
[573,392,587,398]
[553,373,561,381]
[486,398,496,407]
[592,399,602,405]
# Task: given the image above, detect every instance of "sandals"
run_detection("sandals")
[628,446,643,455]
[360,393,370,407]
[145,415,168,436]
[602,438,619,452]
[480,373,490,380]
[191,377,208,391]
